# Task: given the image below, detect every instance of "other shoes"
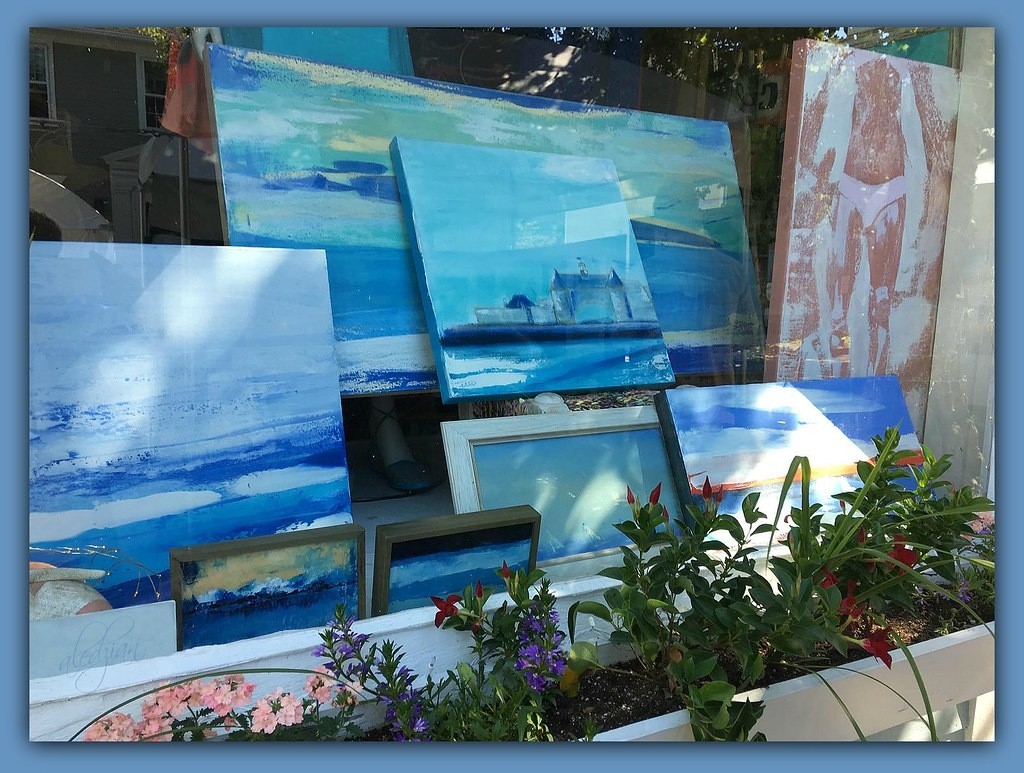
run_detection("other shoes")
[367,402,435,492]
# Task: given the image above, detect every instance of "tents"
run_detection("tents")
[29,169,114,242]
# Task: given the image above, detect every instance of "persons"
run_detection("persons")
[369,396,433,492]
[28,210,62,241]
[642,183,758,387]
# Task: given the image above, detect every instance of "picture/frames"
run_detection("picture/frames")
[170,523,366,652]
[371,504,542,618]
[440,406,687,584]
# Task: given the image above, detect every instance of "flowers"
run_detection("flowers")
[29,417,995,741]
